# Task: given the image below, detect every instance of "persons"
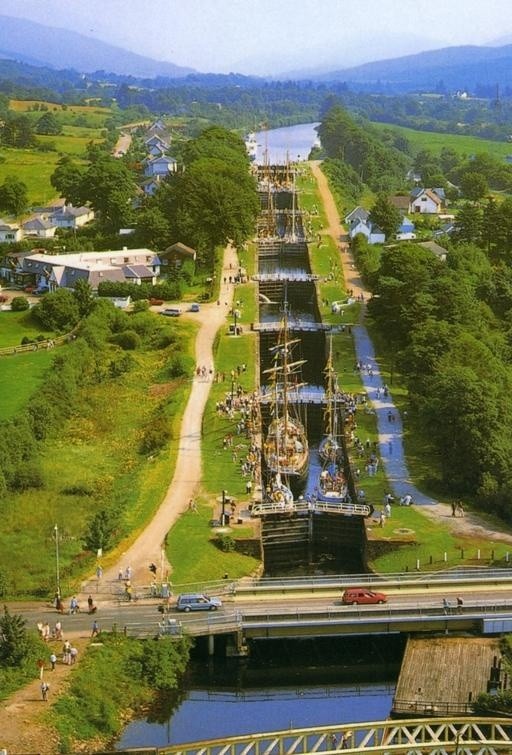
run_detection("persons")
[452,501,464,518]
[36,563,166,702]
[189,499,194,512]
[197,151,413,529]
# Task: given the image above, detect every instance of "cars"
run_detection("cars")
[344,587,388,607]
[163,308,181,317]
[178,591,224,614]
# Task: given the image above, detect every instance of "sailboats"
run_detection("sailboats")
[320,335,348,501]
[253,147,317,246]
[261,295,309,511]
